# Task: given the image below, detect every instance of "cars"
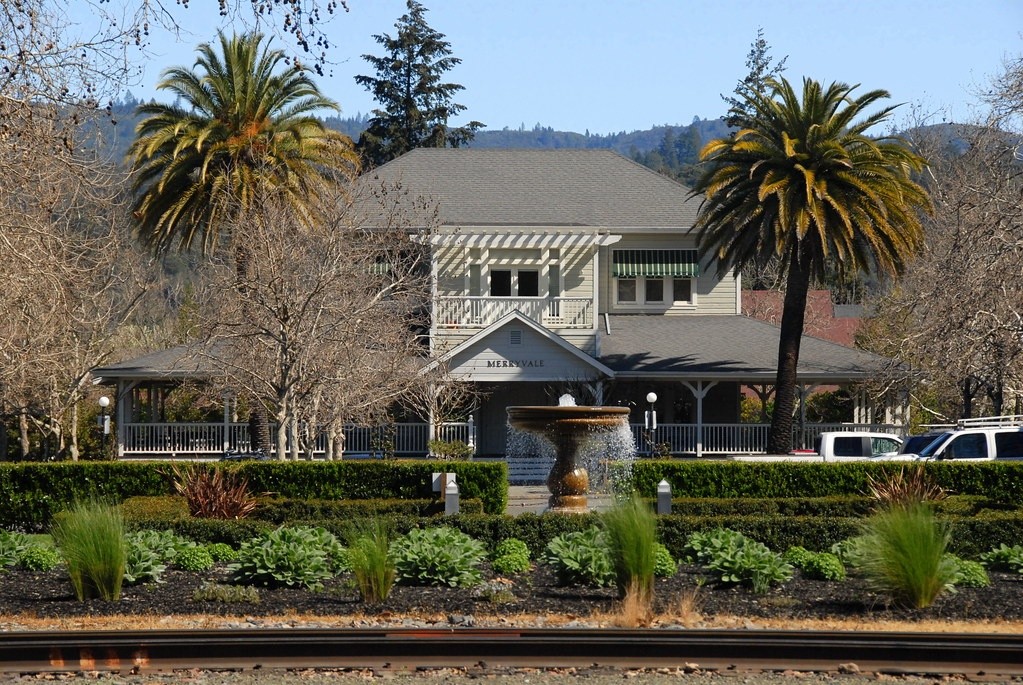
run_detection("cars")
[865,415,1023,463]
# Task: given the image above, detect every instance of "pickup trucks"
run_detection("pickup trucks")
[725,430,905,464]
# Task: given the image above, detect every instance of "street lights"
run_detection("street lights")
[647,391,658,459]
[98,396,110,461]
